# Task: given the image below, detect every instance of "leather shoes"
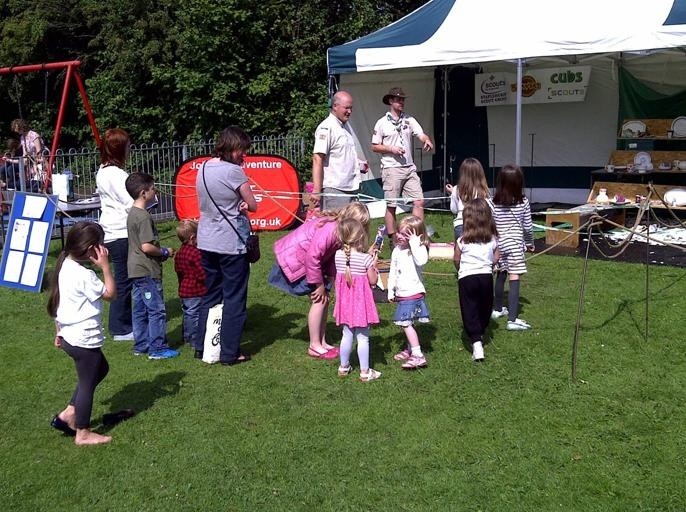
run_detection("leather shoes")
[221,352,251,365]
[51,414,76,436]
[308,346,339,360]
[103,409,135,424]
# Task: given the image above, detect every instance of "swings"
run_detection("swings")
[9,71,51,194]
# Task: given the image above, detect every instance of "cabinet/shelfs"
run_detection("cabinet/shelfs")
[587,116,686,211]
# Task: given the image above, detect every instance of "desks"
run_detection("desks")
[1,199,101,256]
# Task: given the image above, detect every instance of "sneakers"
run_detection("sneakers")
[394,349,428,368]
[506,318,532,330]
[471,341,485,362]
[490,307,509,320]
[113,331,201,360]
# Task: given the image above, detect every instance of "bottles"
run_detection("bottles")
[672,197,677,207]
[62,169,74,202]
[597,189,608,201]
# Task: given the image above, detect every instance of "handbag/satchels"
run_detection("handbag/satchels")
[202,299,225,365]
[246,235,261,263]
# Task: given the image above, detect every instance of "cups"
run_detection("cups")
[667,130,674,139]
[627,164,653,174]
[636,195,649,203]
[605,165,615,173]
[658,160,686,171]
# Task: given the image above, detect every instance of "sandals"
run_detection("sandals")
[338,364,354,377]
[360,369,382,382]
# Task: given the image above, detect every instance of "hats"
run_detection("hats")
[383,88,410,105]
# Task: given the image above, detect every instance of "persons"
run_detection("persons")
[192,125,258,365]
[370,86,435,244]
[331,217,384,382]
[173,218,208,349]
[444,157,493,242]
[0,118,56,218]
[44,220,118,446]
[266,201,372,360]
[93,127,160,342]
[385,215,432,370]
[453,197,501,361]
[124,172,181,361]
[484,163,538,331]
[308,90,370,213]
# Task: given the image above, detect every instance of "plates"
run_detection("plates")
[664,188,686,206]
[651,201,662,205]
[610,199,631,204]
[615,166,626,169]
[622,121,646,138]
[671,117,686,137]
[634,152,651,165]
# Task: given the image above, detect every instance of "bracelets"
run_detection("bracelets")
[161,247,169,261]
